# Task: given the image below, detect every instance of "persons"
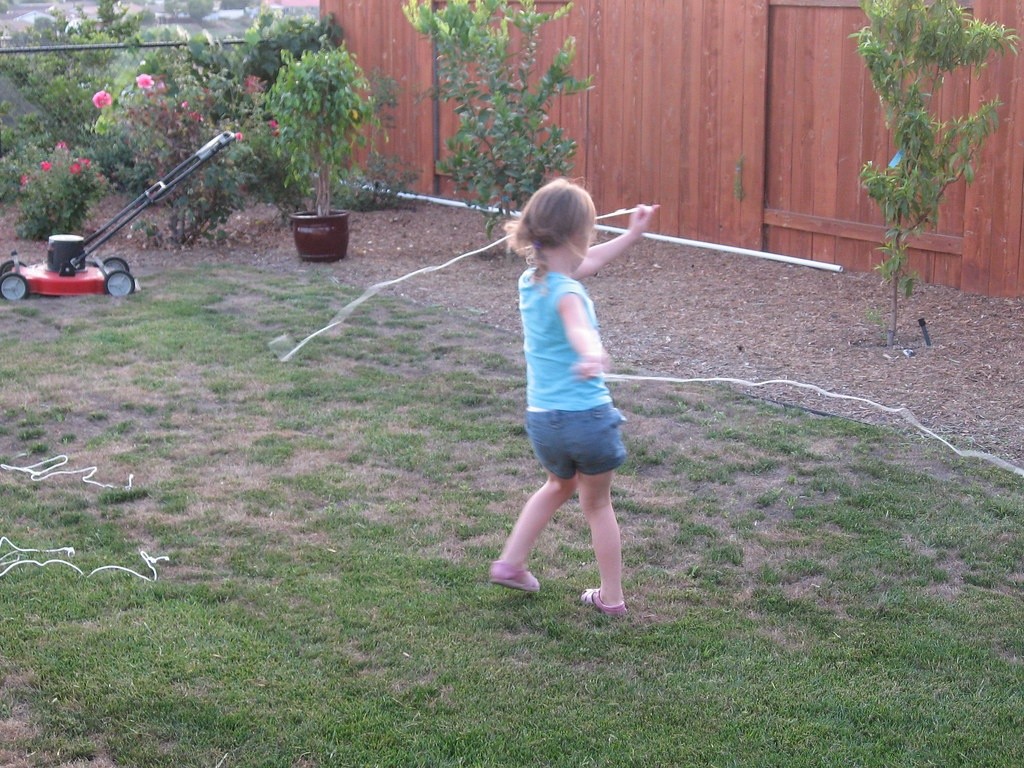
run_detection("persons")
[488,177,661,617]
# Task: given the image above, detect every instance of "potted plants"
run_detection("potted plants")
[265,33,388,264]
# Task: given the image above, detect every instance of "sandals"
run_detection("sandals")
[490,561,540,592]
[580,587,626,616]
